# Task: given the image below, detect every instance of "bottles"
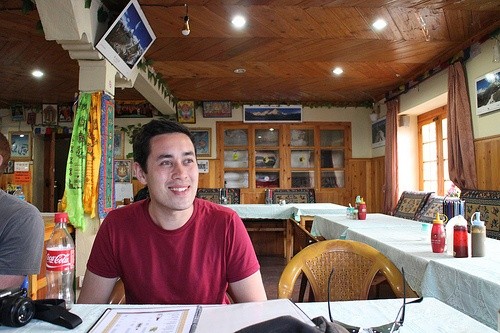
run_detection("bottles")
[358,197,366,220]
[45,212,75,310]
[470,212,486,257]
[453,215,468,258]
[12,187,25,201]
[431,212,448,253]
[347,208,358,220]
[279,198,286,206]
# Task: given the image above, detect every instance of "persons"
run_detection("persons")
[0,133,44,290]
[133,185,198,204]
[76,122,268,305]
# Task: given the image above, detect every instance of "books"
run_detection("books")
[85,298,316,333]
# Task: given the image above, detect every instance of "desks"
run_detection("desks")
[214,202,358,265]
[0,297,500,333]
[311,213,500,333]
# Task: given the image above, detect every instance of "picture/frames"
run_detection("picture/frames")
[202,100,232,118]
[176,99,196,124]
[114,159,132,183]
[8,131,34,161]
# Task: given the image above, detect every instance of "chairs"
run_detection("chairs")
[277,238,419,302]
[289,218,326,303]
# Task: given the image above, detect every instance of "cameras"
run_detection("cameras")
[0,286,35,327]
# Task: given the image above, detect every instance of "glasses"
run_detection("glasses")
[328,267,406,333]
